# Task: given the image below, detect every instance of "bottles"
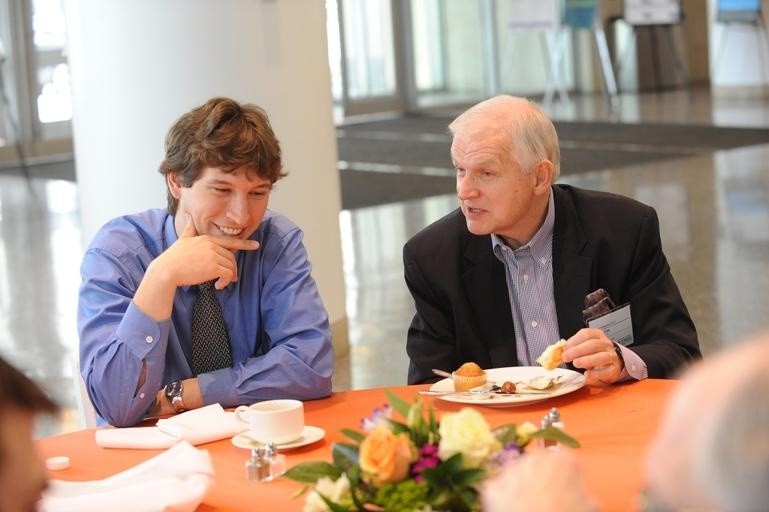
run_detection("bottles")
[243,442,288,483]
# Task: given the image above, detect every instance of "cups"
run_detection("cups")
[235,398,306,447]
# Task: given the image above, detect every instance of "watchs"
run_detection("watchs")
[164,377,187,414]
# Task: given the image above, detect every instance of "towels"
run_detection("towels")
[94,401,244,452]
[31,440,216,512]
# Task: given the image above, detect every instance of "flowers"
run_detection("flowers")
[278,377,584,511]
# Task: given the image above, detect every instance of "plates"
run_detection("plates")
[227,425,326,451]
[428,365,587,408]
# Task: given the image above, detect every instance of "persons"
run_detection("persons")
[403,94,703,396]
[77,97,333,428]
[0,359,60,511]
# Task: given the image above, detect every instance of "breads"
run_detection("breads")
[537,338,567,370]
[454,361,487,392]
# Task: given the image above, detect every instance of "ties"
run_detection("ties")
[190,277,234,379]
